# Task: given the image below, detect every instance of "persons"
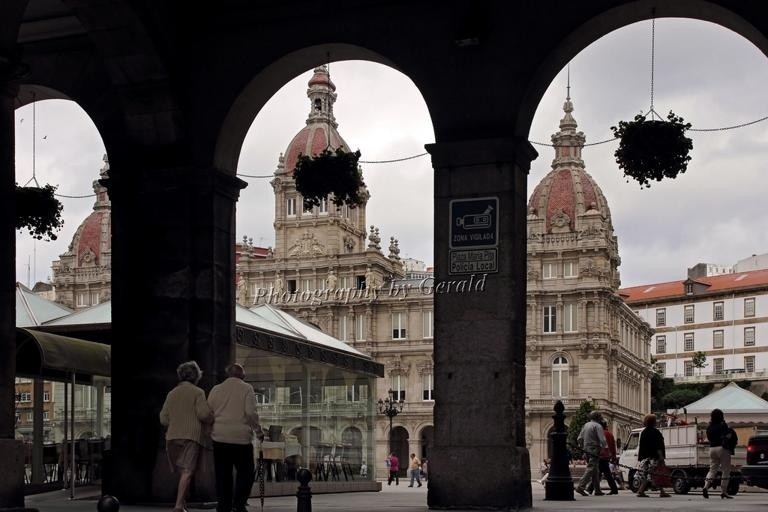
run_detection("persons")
[420,456,429,482]
[326,270,337,292]
[236,275,248,307]
[407,453,422,487]
[159,359,214,512]
[364,268,375,290]
[274,273,285,298]
[387,452,400,485]
[635,414,673,497]
[384,452,392,481]
[360,460,368,479]
[702,408,736,498]
[535,407,629,499]
[203,363,265,512]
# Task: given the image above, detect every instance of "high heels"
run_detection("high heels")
[720,493,733,499]
[702,487,709,498]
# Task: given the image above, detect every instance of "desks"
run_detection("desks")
[256,441,300,482]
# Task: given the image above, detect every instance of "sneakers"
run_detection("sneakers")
[637,491,650,496]
[408,483,422,487]
[660,492,672,498]
[576,486,618,496]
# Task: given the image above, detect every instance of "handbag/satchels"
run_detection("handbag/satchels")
[650,464,673,487]
[721,428,737,450]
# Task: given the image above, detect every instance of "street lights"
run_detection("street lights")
[378,387,404,452]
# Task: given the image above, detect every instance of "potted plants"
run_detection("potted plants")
[614,1,692,189]
[291,45,369,213]
[10,92,60,243]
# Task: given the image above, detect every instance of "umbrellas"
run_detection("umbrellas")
[256,439,266,512]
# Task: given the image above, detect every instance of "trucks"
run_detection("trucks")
[616,424,746,496]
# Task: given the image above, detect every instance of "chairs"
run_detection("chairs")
[310,443,355,482]
[23,438,109,486]
